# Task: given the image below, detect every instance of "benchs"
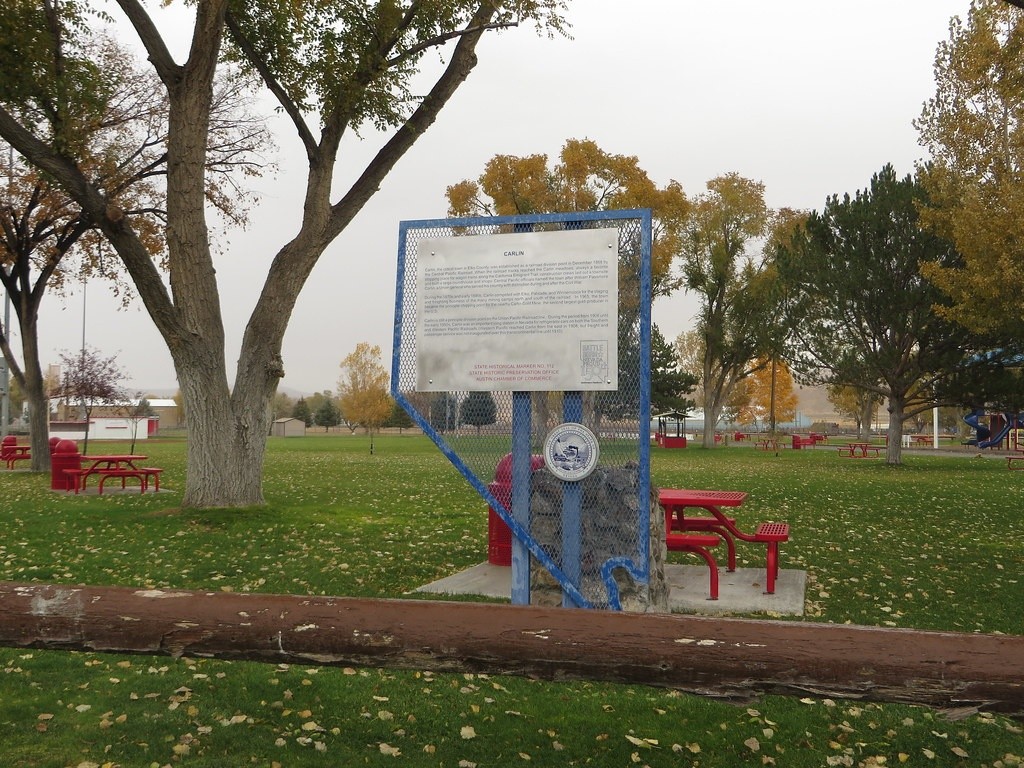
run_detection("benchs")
[143,468,163,492]
[886,433,934,447]
[83,467,127,489]
[10,454,32,470]
[665,533,721,600]
[713,431,827,449]
[1016,449,1024,454]
[863,448,880,457]
[1006,455,1024,471]
[97,470,147,495]
[662,516,737,571]
[838,448,852,455]
[754,521,789,595]
[62,468,83,495]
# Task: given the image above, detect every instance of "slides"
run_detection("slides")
[962,410,990,445]
[979,424,1012,448]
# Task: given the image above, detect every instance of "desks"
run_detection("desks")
[849,443,871,456]
[3,446,32,467]
[658,487,747,532]
[81,455,149,490]
